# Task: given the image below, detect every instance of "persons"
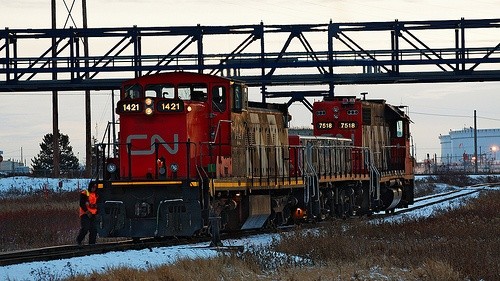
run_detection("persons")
[76,180,100,247]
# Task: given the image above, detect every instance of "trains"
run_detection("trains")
[90,71,418,238]
